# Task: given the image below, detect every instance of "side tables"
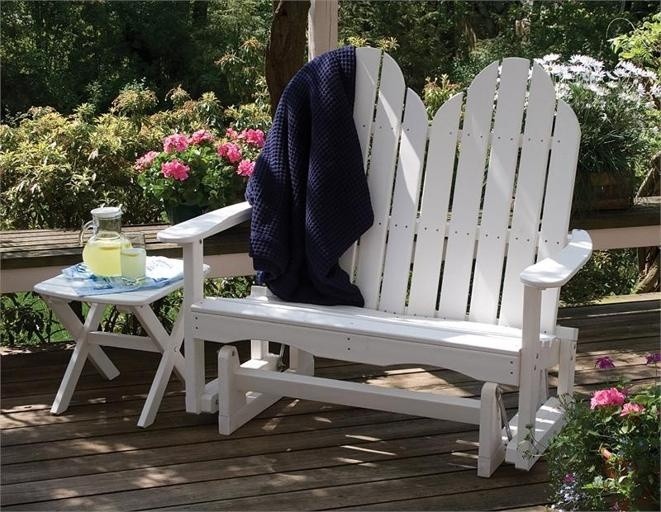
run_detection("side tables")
[35,257,207,428]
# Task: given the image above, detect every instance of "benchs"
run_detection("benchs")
[155,46,592,478]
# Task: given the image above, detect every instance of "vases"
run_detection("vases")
[569,169,635,213]
[164,195,203,223]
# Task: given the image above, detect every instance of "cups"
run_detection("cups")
[120,232,146,283]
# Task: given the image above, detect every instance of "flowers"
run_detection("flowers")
[134,130,263,201]
[494,54,661,172]
